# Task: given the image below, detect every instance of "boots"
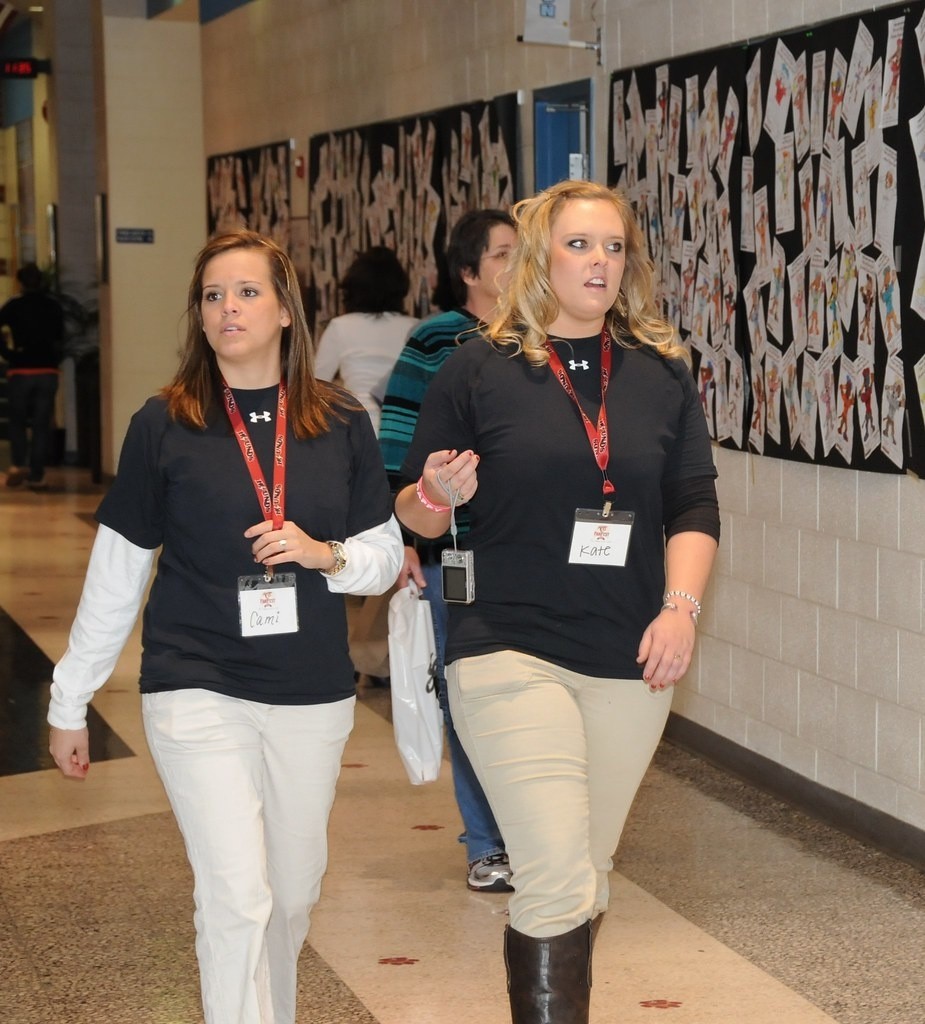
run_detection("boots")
[504,911,609,1024]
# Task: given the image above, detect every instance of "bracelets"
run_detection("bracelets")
[416,476,450,513]
[662,591,701,627]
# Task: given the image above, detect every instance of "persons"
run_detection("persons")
[0,266,65,489]
[379,207,521,891]
[393,184,722,1024]
[46,232,404,1024]
[312,245,420,444]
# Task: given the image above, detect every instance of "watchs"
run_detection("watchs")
[318,542,347,576]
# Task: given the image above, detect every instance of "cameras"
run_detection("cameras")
[441,548,475,605]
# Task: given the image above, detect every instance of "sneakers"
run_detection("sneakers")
[467,853,515,892]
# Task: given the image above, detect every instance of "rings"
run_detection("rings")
[280,540,287,551]
[675,655,682,661]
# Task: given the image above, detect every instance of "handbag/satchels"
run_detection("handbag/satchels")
[383,579,444,786]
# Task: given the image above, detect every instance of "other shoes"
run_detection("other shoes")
[23,477,46,490]
[6,467,21,486]
